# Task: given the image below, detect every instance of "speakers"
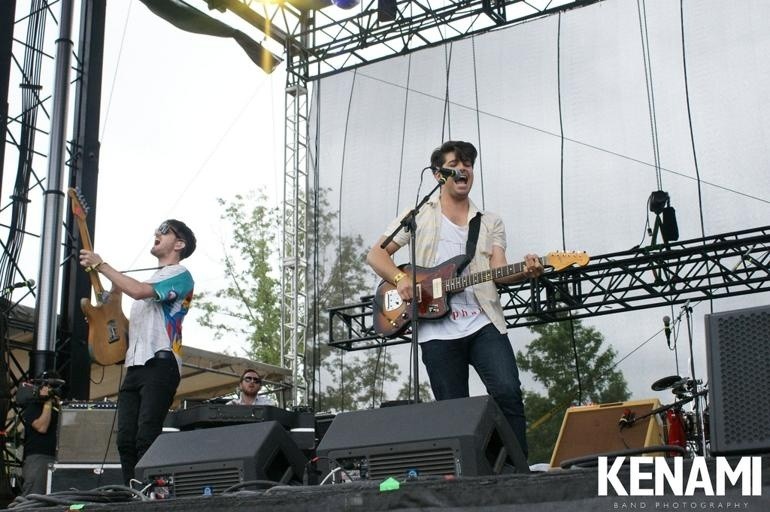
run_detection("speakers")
[550,396,666,472]
[314,395,532,485]
[55,402,122,468]
[134,420,302,498]
[704,305,770,456]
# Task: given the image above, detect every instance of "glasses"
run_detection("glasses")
[158,222,181,242]
[239,376,262,386]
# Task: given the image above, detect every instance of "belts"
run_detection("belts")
[153,350,174,360]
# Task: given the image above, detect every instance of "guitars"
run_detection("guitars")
[373,250,590,339]
[67,187,129,366]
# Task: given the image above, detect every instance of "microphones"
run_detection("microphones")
[6,279,35,291]
[431,165,462,181]
[663,316,672,345]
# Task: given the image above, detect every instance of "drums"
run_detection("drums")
[666,408,693,458]
[652,376,682,391]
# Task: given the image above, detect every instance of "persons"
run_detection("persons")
[20,370,68,501]
[79,219,197,486]
[367,141,546,470]
[225,368,278,406]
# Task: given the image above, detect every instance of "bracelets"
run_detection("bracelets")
[44,401,53,409]
[393,271,407,286]
